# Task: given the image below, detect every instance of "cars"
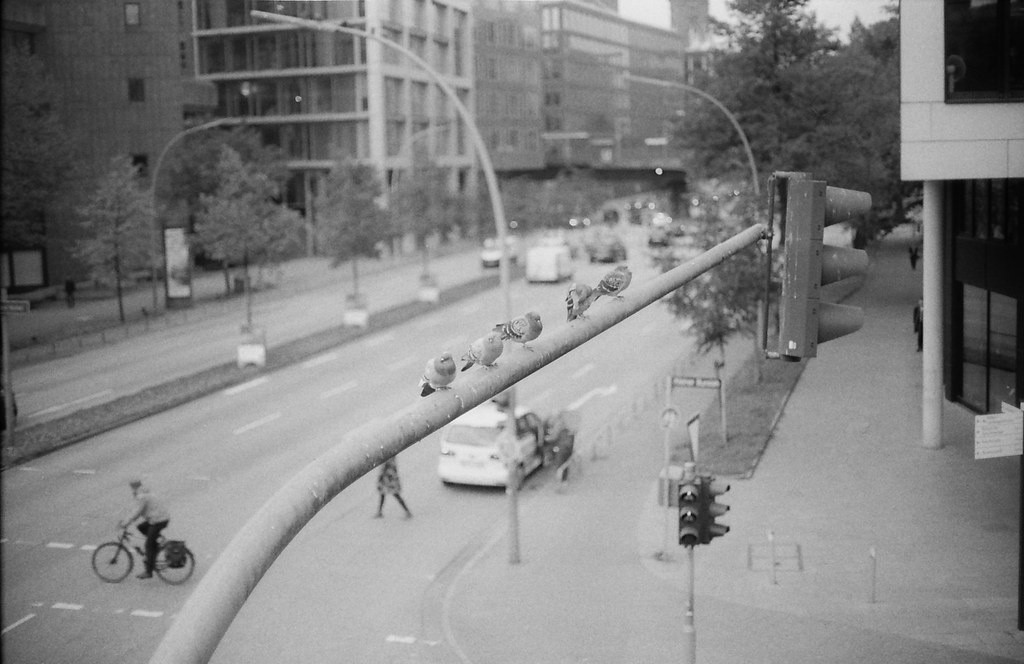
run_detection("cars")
[482,212,684,284]
[440,401,545,490]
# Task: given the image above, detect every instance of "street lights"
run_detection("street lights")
[149,117,243,315]
[247,8,519,564]
[618,73,761,390]
[382,126,450,266]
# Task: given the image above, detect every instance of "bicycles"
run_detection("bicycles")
[92,523,195,585]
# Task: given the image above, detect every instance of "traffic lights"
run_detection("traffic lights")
[776,170,873,360]
[678,478,732,546]
[492,396,509,407]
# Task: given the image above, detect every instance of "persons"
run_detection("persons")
[375,454,410,518]
[908,240,918,270]
[913,297,923,352]
[117,479,170,579]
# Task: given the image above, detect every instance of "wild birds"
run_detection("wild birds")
[492,311,543,348]
[418,352,456,397]
[593,265,632,301]
[461,332,503,372]
[565,283,594,322]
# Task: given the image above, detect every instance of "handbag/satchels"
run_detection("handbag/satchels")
[162,541,186,569]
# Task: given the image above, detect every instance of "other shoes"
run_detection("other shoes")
[135,571,154,579]
